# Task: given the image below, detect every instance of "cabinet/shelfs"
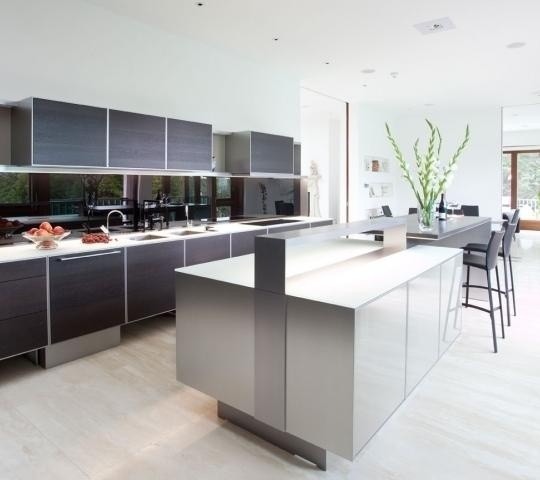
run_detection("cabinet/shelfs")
[32,94,108,170]
[126,240,186,324]
[231,227,268,259]
[48,248,125,346]
[438,251,464,362]
[406,264,441,407]
[0,258,49,360]
[108,107,166,170]
[250,131,293,175]
[166,117,213,171]
[269,223,313,236]
[185,232,230,268]
[353,284,407,463]
[312,220,332,229]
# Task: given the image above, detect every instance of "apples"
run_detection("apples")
[53,226,63,234]
[29,227,34,234]
[40,221,52,233]
[36,229,46,236]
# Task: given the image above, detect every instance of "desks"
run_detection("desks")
[358,213,493,302]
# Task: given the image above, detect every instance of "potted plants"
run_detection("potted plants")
[385,118,470,232]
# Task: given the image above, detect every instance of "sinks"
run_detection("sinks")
[170,229,205,236]
[124,233,167,242]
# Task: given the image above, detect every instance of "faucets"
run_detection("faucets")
[184,204,193,229]
[99,210,127,234]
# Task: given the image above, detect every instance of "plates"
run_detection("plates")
[21,231,72,250]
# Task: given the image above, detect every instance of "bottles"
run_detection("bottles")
[438,193,446,220]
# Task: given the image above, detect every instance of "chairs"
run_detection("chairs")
[445,206,452,214]
[453,209,462,216]
[500,206,521,241]
[382,205,392,217]
[407,206,418,215]
[459,228,506,353]
[466,221,518,325]
[460,205,479,217]
[502,212,521,234]
